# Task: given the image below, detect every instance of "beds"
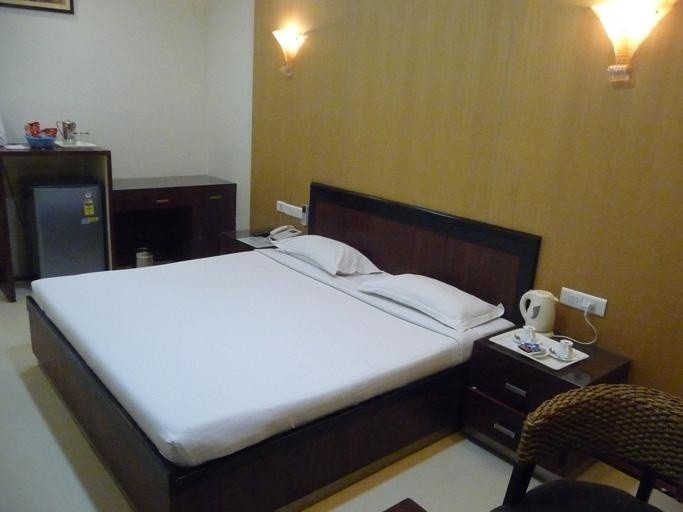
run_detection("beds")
[25,182,543,512]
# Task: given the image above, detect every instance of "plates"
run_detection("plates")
[548,346,575,362]
[513,331,550,358]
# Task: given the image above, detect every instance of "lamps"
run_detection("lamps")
[272,29,306,78]
[591,0,674,90]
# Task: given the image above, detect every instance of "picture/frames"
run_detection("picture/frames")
[0,0,74,15]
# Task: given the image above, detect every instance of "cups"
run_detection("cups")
[558,339,575,359]
[69,131,81,144]
[134,246,155,267]
[522,326,538,344]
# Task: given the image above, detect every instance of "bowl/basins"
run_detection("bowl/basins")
[25,135,57,148]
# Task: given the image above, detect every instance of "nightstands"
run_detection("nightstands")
[461,323,630,485]
[218,227,280,254]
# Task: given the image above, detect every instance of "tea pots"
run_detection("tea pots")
[57,120,76,143]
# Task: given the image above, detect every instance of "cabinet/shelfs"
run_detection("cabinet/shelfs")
[0,142,112,302]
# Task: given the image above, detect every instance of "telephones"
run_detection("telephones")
[270,224,303,241]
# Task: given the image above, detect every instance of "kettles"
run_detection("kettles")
[519,288,560,336]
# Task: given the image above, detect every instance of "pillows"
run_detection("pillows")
[358,272,505,332]
[270,235,384,275]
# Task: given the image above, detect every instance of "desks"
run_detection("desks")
[112,174,236,260]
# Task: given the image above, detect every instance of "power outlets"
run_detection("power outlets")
[586,294,607,317]
[276,200,287,212]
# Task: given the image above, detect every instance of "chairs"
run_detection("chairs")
[489,382,682,512]
